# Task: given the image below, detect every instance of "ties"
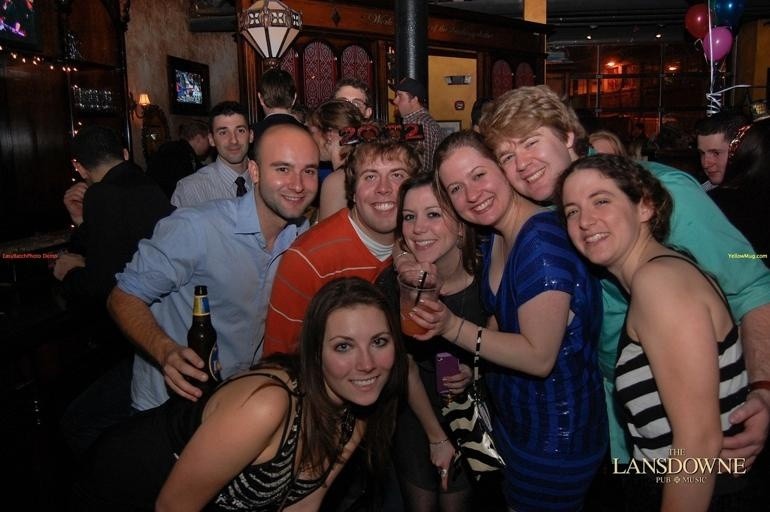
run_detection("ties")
[235,177,247,197]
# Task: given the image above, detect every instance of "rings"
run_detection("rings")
[441,470,447,477]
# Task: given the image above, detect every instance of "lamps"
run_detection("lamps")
[236,0,304,69]
[129,91,150,121]
[723,98,770,123]
[513,0,547,25]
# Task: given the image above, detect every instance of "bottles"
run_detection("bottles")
[187,285,219,393]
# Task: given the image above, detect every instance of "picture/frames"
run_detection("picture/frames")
[434,120,462,136]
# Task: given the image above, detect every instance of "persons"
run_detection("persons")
[332,76,376,121]
[476,84,770,511]
[260,134,421,361]
[409,128,609,511]
[105,120,322,512]
[0,127,179,512]
[308,98,374,228]
[149,115,211,195]
[373,168,505,511]
[171,100,260,209]
[116,276,408,512]
[245,68,314,160]
[470,94,496,132]
[591,106,755,192]
[556,152,770,511]
[390,78,445,175]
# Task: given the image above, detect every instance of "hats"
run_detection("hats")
[388,77,426,97]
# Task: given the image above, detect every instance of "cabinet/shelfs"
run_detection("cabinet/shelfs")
[544,34,737,147]
[238,23,394,139]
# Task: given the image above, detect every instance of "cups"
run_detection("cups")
[397,269,445,337]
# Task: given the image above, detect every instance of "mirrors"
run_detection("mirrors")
[141,104,172,164]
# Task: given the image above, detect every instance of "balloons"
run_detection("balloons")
[709,0,746,29]
[703,27,732,63]
[684,4,711,38]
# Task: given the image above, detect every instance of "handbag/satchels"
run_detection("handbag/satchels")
[441,375,512,487]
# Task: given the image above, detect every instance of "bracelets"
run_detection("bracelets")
[392,251,408,267]
[745,380,770,395]
[451,316,467,344]
[429,438,449,445]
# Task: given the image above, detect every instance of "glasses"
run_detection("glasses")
[345,99,367,107]
